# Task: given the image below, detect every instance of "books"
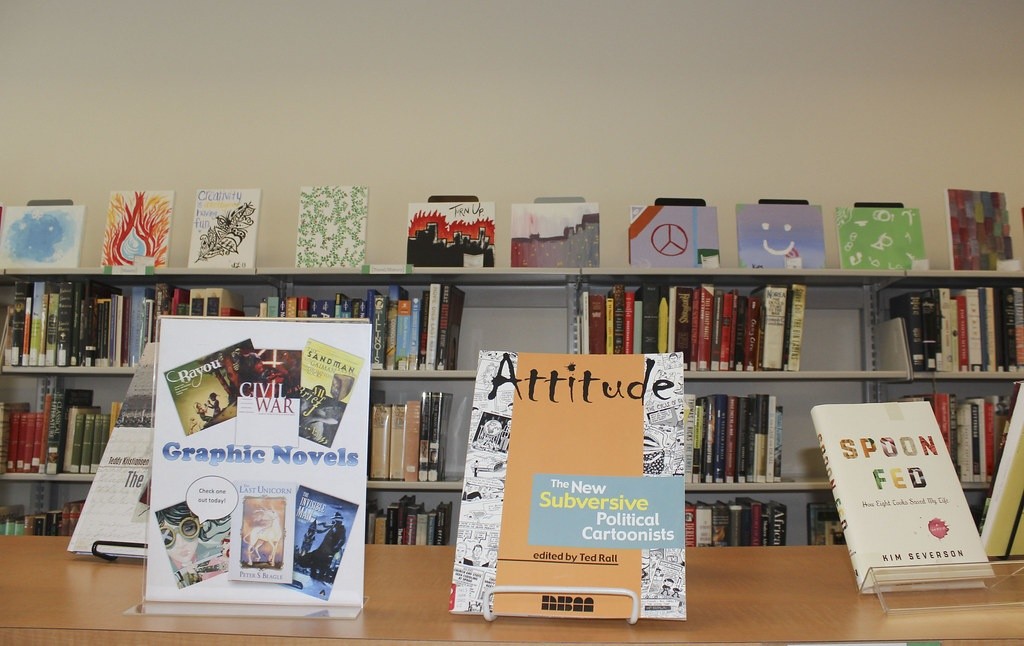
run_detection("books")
[0,182,1023,622]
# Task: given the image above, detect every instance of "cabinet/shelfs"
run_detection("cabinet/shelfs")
[0,267,1020,547]
